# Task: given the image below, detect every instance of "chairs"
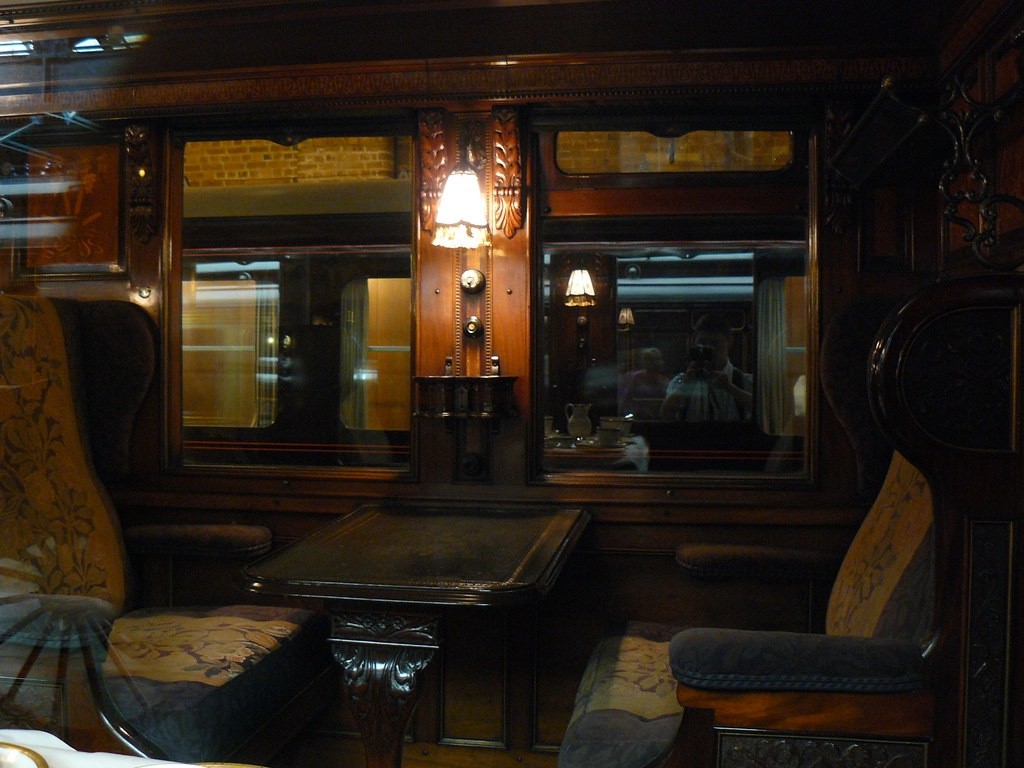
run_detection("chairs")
[0,289,329,765]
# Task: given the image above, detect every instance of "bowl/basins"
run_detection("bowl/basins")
[596,429,621,447]
[600,417,634,438]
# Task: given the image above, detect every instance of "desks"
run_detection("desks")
[238,502,595,768]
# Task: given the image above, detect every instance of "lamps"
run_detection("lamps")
[426,121,496,250]
[618,307,636,333]
[552,270,1024,768]
[565,268,595,309]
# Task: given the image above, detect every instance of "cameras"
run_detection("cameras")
[688,345,713,363]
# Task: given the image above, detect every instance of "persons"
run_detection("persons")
[659,312,753,420]
[621,348,669,418]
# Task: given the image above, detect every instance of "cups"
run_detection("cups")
[544,416,553,433]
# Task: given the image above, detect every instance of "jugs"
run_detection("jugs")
[565,403,591,439]
